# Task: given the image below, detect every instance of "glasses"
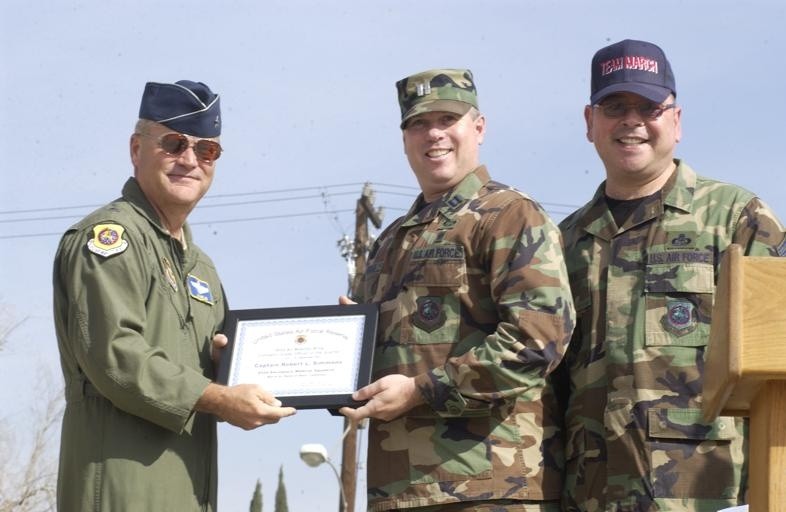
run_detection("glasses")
[594,102,674,120]
[141,130,223,163]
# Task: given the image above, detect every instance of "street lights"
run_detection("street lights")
[298,443,349,512]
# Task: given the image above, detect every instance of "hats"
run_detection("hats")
[590,37,676,107]
[137,78,223,139]
[395,67,479,129]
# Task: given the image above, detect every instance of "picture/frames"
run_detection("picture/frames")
[216,306,380,408]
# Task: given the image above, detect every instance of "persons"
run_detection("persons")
[556,37,786,511]
[336,68,578,512]
[52,78,295,512]
